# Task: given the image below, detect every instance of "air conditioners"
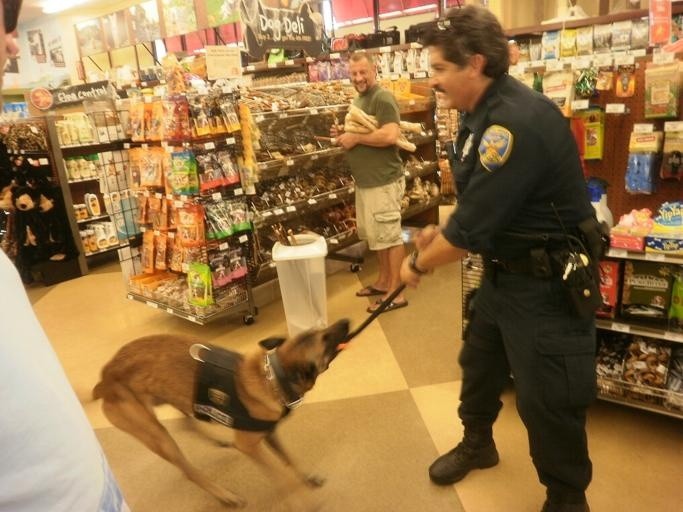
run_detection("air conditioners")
[91,318,350,509]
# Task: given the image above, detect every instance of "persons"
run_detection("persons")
[329,51,407,312]
[1,0,134,512]
[396,2,610,509]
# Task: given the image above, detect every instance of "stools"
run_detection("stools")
[487,249,562,273]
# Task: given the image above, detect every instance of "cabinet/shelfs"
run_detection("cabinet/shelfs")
[446,0,683,421]
[0,2,449,327]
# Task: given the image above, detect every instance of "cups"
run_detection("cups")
[433,15,470,58]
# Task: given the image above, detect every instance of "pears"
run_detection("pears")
[587,175,614,230]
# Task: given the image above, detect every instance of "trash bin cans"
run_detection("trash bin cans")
[407,250,430,277]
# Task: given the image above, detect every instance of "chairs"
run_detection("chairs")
[540,501,589,512]
[428,439,498,485]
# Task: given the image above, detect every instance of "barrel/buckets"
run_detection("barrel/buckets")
[367,298,407,313]
[355,286,386,296]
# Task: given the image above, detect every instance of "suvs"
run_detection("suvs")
[272,233,330,340]
